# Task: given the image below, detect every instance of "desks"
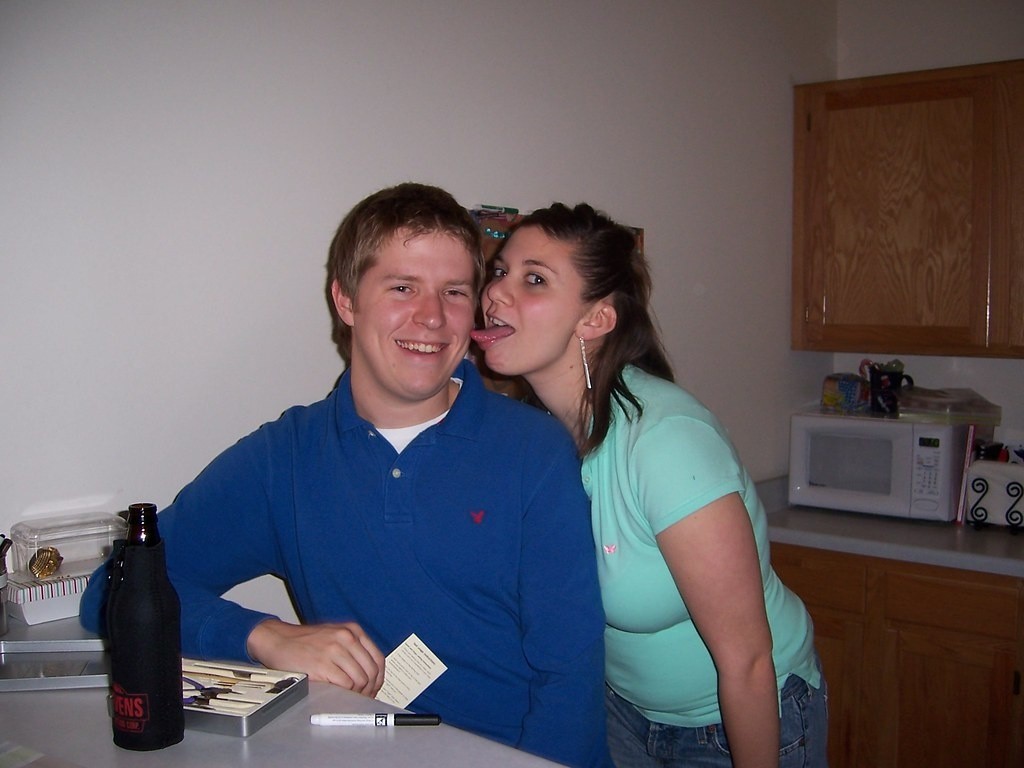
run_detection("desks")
[0,610,566,768]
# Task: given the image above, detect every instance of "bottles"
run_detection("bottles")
[112,503,185,752]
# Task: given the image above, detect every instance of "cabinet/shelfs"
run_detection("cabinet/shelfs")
[769,541,1024,768]
[792,59,1024,359]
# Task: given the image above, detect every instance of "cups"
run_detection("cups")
[870,371,914,419]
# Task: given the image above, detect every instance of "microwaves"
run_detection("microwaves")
[788,403,994,522]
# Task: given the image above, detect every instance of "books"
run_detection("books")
[957,424,974,526]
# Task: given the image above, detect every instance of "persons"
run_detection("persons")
[469,202,830,768]
[79,179,611,768]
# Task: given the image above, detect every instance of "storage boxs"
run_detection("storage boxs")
[9,511,132,582]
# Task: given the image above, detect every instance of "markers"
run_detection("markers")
[309,710,440,727]
